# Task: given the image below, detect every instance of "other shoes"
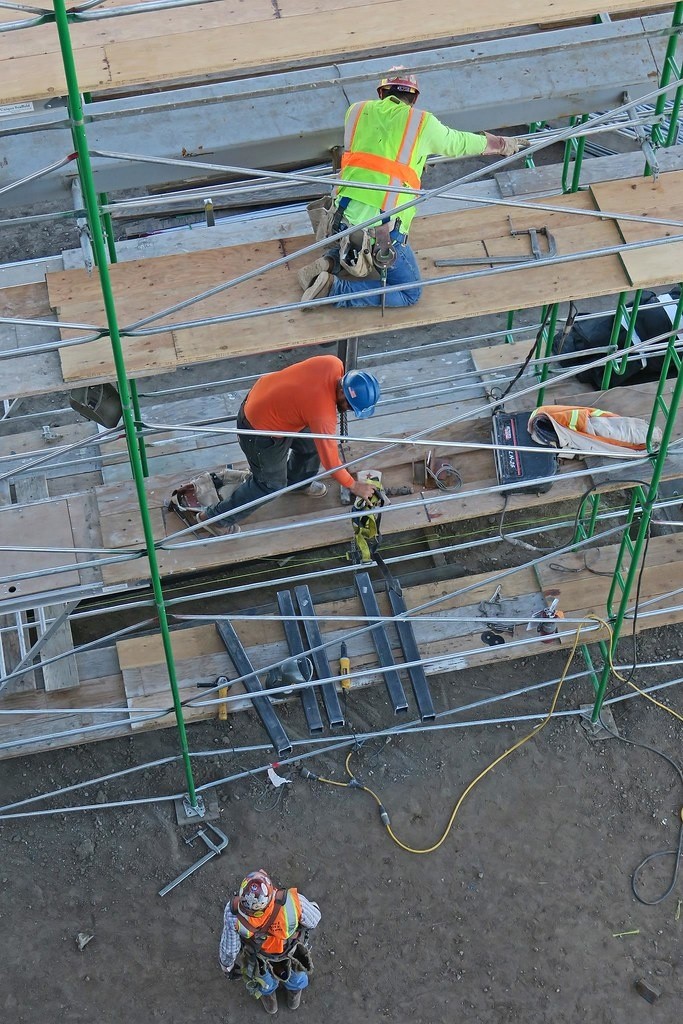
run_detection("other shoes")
[299,256,333,290]
[299,271,335,311]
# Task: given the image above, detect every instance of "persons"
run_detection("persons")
[298,65,530,308]
[217,870,320,1016]
[196,356,382,540]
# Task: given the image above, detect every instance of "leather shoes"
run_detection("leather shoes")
[197,510,241,538]
[286,479,327,498]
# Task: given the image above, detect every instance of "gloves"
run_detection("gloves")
[481,131,530,157]
[224,964,242,981]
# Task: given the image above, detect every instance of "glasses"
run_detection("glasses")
[346,398,355,411]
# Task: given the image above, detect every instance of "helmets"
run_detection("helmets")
[341,369,380,417]
[239,871,272,916]
[377,67,419,95]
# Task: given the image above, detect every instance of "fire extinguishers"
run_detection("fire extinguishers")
[536,599,565,643]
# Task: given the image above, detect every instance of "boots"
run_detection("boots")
[287,989,302,1010]
[258,991,278,1014]
